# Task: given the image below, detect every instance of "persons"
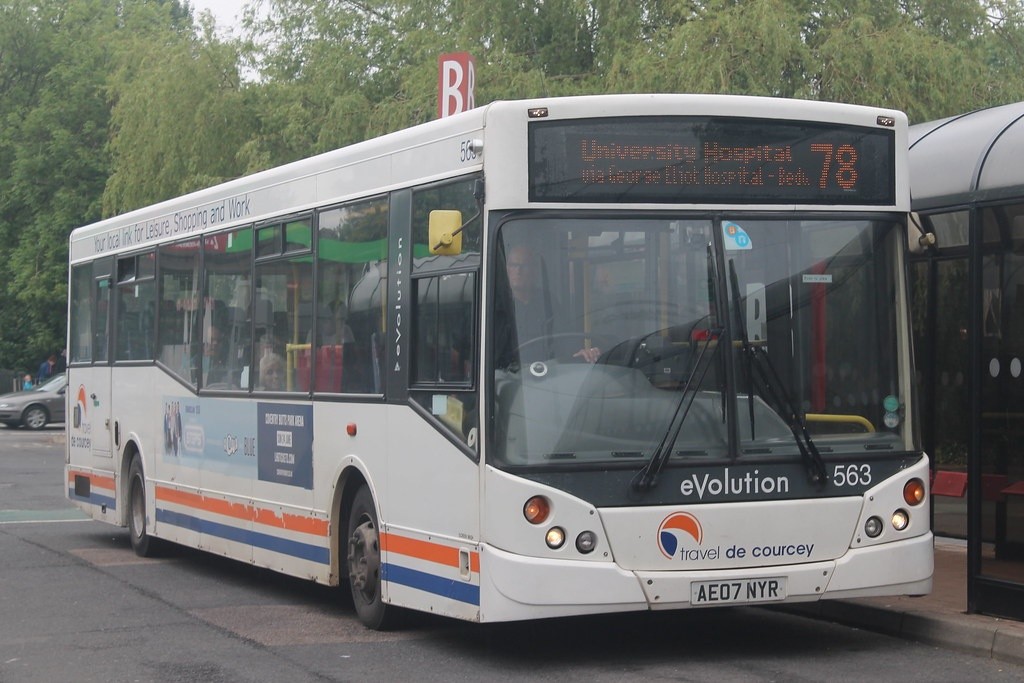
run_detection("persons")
[37,354,56,385]
[460,241,601,370]
[195,298,355,394]
[23,375,33,391]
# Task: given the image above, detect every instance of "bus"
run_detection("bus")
[64,90,940,631]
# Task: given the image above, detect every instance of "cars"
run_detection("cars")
[0,371,68,431]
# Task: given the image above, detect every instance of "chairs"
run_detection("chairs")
[116,299,476,392]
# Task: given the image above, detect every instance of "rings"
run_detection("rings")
[590,349,594,350]
